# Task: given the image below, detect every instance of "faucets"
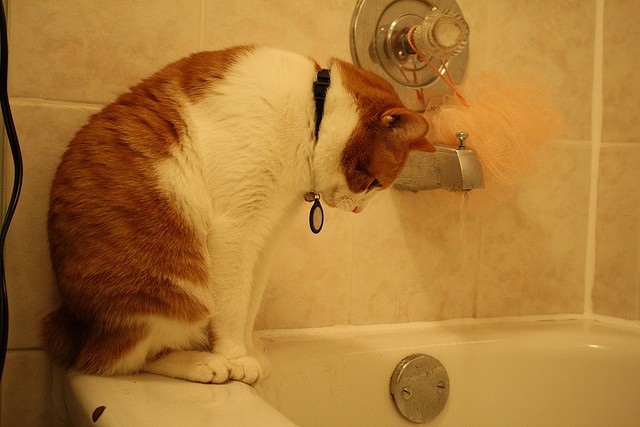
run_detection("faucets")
[392,131,485,192]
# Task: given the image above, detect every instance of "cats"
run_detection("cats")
[40,45,436,387]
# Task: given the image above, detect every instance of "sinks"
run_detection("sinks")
[54,316,638,426]
